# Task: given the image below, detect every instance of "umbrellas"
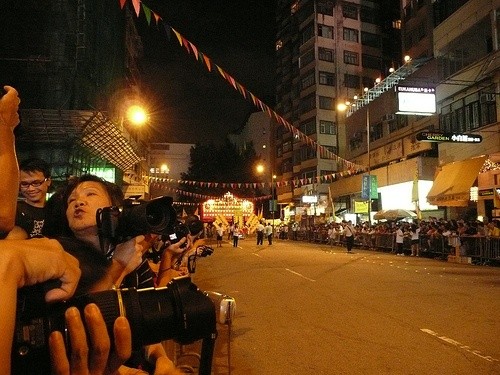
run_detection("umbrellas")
[373,210,417,221]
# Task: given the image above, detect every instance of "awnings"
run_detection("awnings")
[425,158,487,208]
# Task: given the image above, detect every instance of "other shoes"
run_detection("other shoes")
[397,253,405,256]
[410,254,414,256]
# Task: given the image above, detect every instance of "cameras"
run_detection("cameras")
[10,273,216,375]
[102,194,214,264]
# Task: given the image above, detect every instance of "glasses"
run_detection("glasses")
[19,178,47,188]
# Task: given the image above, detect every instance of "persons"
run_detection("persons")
[0,84,500,375]
